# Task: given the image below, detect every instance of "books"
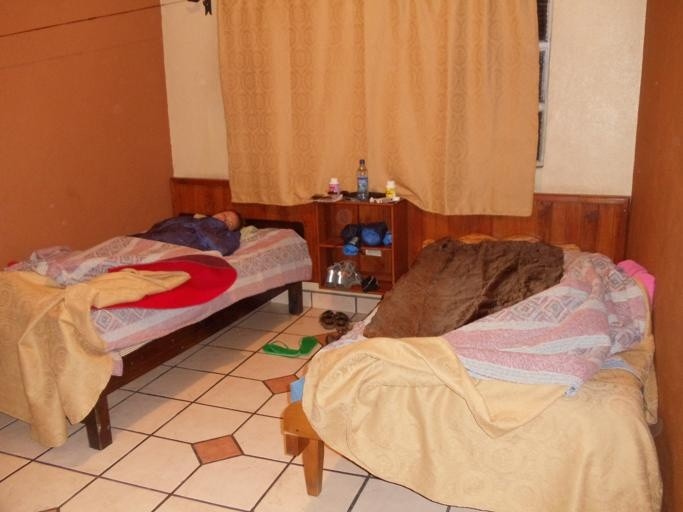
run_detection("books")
[307,192,343,203]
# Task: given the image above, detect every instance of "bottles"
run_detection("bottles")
[385,179,396,201]
[355,159,370,202]
[328,177,340,198]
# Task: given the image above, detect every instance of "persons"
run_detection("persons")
[186,207,244,233]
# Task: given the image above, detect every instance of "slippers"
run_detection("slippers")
[262,340,300,358]
[300,336,317,354]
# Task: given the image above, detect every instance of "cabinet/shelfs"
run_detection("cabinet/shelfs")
[313,197,408,296]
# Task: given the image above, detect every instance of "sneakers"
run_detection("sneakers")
[325,261,362,288]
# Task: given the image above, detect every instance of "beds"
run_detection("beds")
[0,213,304,451]
[282,234,652,498]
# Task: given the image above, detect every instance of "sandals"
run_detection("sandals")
[334,312,348,330]
[320,310,335,329]
